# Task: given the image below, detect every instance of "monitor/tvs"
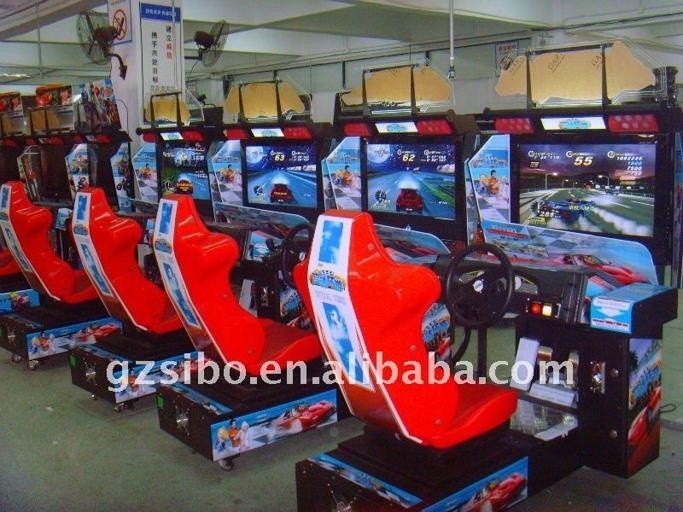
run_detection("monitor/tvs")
[88,149,118,190]
[240,140,322,211]
[44,151,71,198]
[509,136,661,240]
[156,143,210,205]
[360,135,457,225]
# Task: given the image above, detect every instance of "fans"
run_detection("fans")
[75,9,127,81]
[183,21,228,66]
